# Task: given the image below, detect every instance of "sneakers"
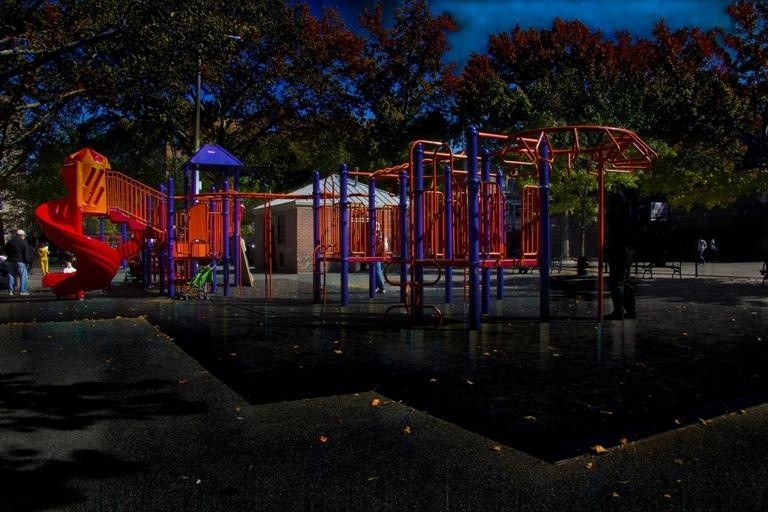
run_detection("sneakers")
[624,310,638,319]
[604,311,624,320]
[18,292,30,296]
[8,289,16,296]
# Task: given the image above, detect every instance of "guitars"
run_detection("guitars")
[35,193,122,300]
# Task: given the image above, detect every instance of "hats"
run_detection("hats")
[16,229,27,236]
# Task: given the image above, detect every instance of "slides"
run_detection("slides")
[230,237,254,288]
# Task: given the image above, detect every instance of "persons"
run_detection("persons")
[696,236,708,265]
[710,235,718,263]
[56,247,78,274]
[371,219,390,293]
[599,190,639,322]
[37,242,50,276]
[1,229,30,297]
